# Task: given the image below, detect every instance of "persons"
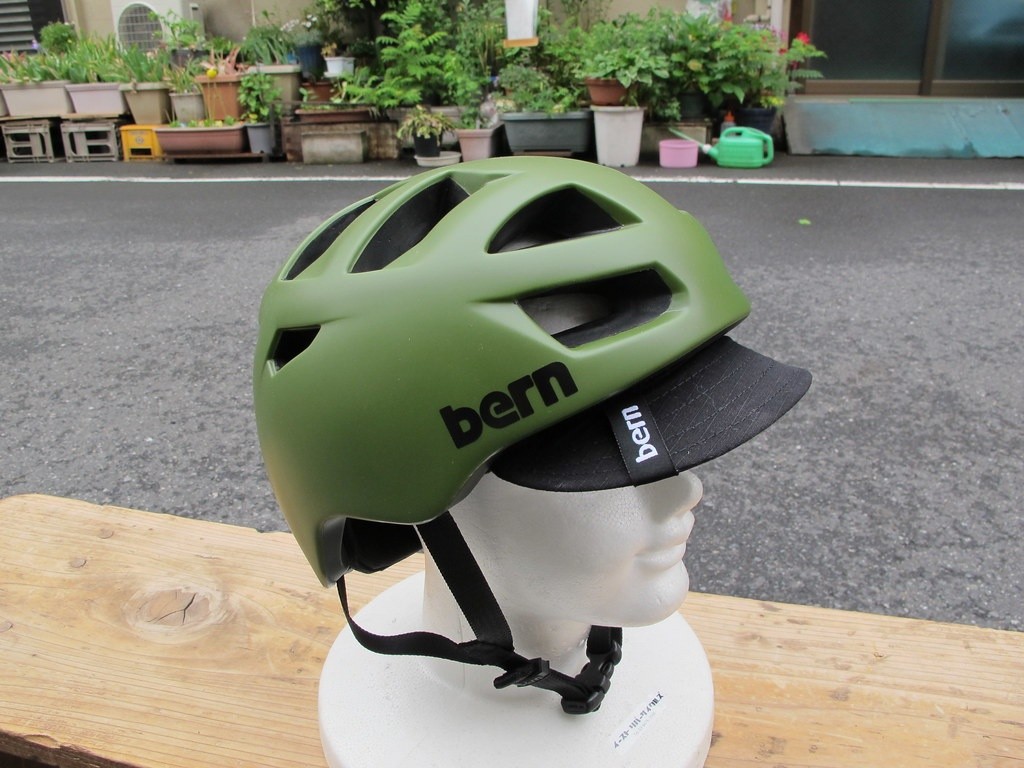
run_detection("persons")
[254,156,813,768]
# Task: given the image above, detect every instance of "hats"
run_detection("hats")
[489,304,812,492]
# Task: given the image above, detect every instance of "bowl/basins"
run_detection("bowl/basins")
[659,139,699,167]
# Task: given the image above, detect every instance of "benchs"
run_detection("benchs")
[0,486,1024,768]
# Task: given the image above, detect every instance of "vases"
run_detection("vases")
[712,102,777,139]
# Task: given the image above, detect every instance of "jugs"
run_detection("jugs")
[668,128,775,168]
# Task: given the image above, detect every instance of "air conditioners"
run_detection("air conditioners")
[110,0,204,62]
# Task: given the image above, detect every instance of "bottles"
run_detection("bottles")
[721,112,736,136]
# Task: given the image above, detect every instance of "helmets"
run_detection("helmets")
[252,155,753,587]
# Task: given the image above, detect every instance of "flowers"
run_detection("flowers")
[712,11,828,139]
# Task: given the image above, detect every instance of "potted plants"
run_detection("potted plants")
[0,0,745,167]
